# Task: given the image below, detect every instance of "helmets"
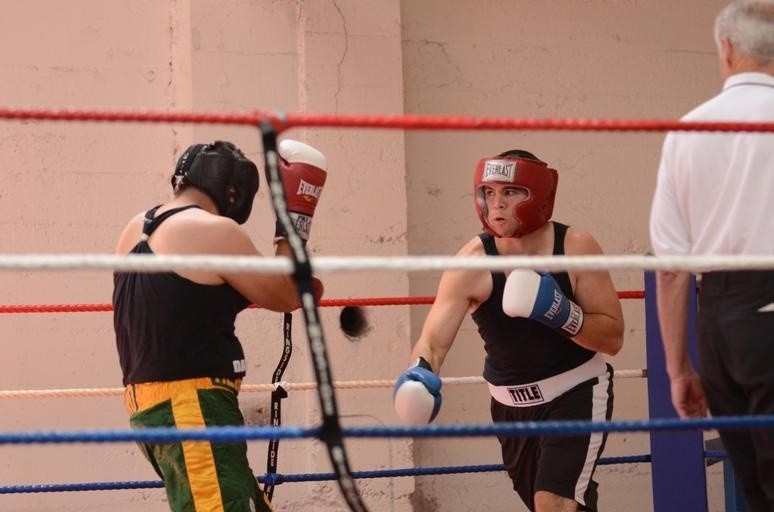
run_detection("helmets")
[171,143,259,226]
[472,157,559,241]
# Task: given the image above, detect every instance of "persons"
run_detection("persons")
[108,139,328,511]
[646,0,773,511]
[387,146,626,512]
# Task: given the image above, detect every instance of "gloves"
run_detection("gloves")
[390,356,444,425]
[496,264,585,340]
[264,137,326,248]
